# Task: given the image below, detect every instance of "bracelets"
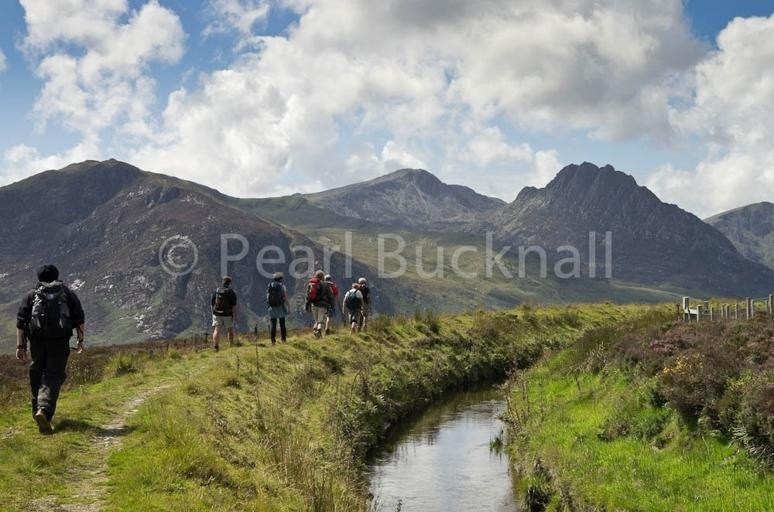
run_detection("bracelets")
[16,345,23,349]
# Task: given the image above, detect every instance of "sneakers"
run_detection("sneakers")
[35,408,52,433]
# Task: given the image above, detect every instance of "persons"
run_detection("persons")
[210,275,241,349]
[306,270,371,338]
[16,264,86,434]
[266,271,292,345]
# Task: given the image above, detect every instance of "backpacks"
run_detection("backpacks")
[213,286,227,314]
[308,278,323,305]
[269,283,284,307]
[29,286,70,336]
[345,288,361,310]
